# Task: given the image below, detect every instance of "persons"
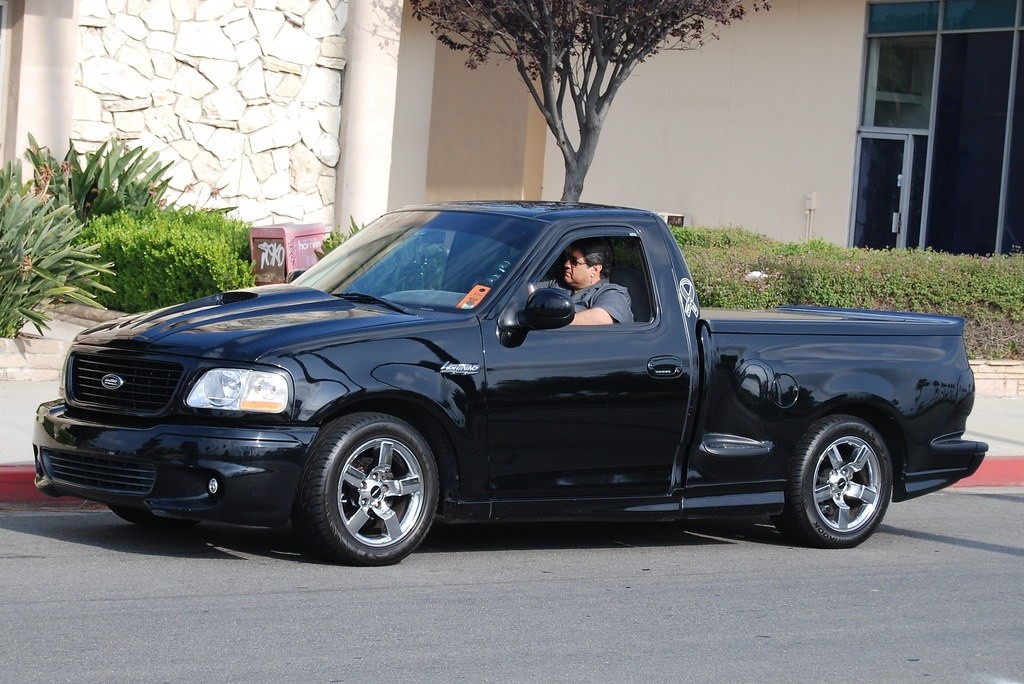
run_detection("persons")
[534,238,634,325]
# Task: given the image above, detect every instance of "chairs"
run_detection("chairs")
[610,265,650,323]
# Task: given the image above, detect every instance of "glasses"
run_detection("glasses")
[568,255,590,266]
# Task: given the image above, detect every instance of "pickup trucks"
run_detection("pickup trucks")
[34,201,989,567]
